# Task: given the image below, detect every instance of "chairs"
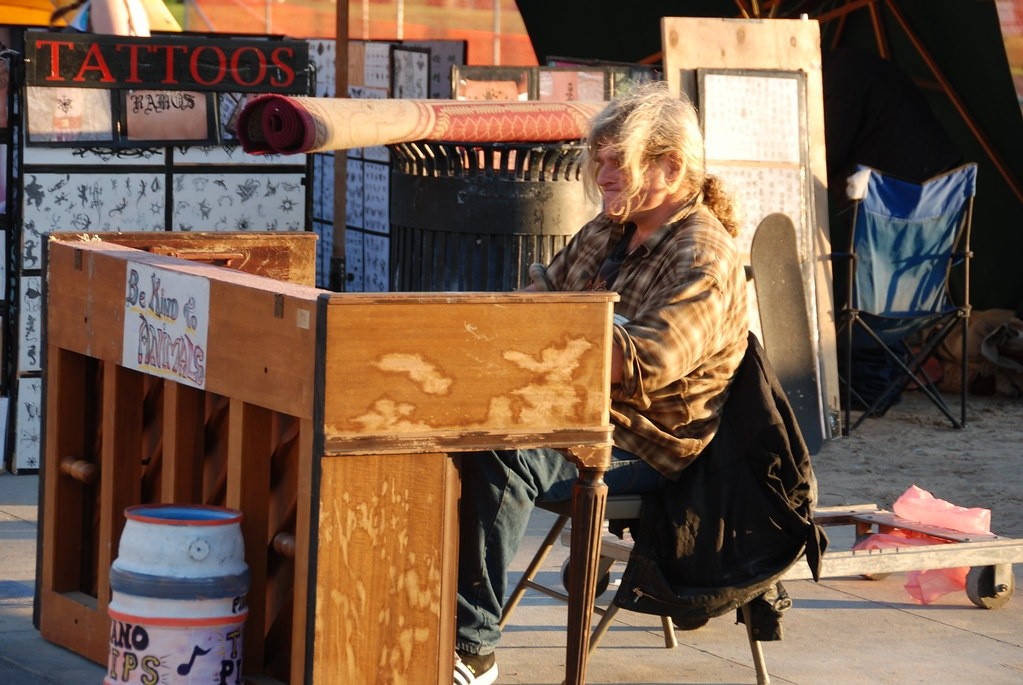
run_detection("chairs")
[830,162,981,439]
[501,485,773,685]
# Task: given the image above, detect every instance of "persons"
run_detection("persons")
[453,71,750,685]
[822,46,972,300]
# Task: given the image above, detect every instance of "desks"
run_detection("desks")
[32,228,623,685]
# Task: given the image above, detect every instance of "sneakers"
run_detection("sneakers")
[454,646,499,685]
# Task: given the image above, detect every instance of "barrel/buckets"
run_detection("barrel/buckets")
[101,501,250,685]
